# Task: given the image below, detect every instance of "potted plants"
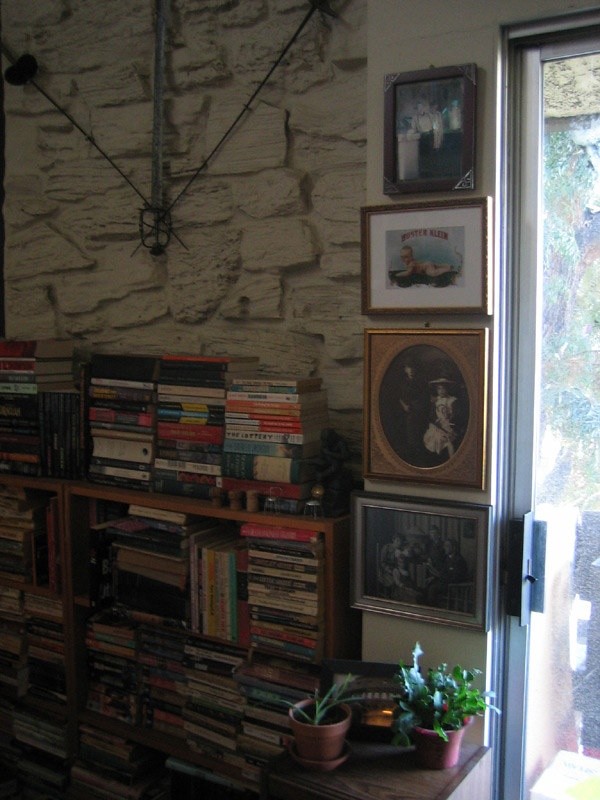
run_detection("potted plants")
[250,675,364,762]
[391,640,502,769]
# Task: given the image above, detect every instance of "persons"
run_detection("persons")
[396,357,457,461]
[409,95,464,176]
[379,523,469,606]
[394,246,457,278]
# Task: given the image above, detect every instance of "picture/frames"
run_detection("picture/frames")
[362,327,492,491]
[360,195,494,317]
[346,488,494,633]
[384,62,479,195]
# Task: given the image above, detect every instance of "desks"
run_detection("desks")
[258,737,494,799]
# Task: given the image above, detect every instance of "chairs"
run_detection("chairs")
[444,581,473,612]
[376,541,395,599]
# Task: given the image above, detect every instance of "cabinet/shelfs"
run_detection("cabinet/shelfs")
[62,478,361,800]
[0,471,74,799]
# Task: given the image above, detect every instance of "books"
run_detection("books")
[0,335,326,800]
